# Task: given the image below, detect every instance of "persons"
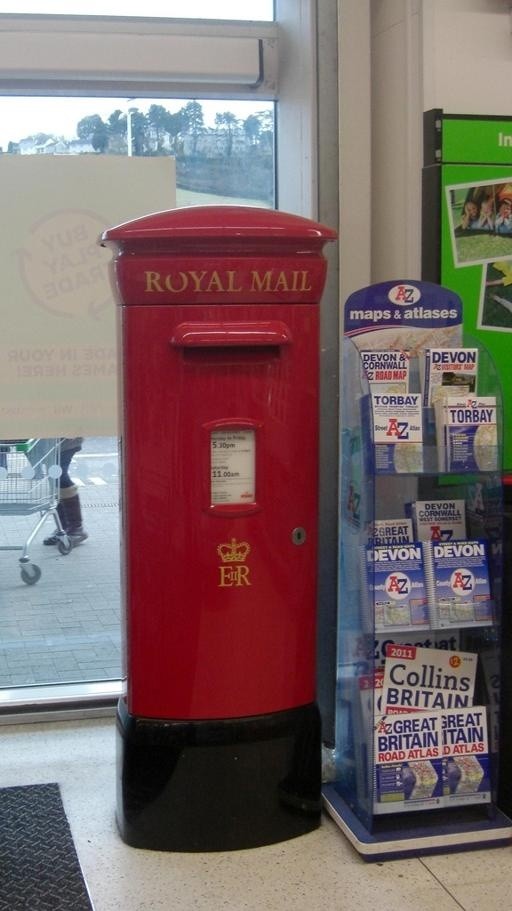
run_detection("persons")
[459,196,478,229]
[21,436,91,549]
[472,195,494,232]
[0,440,11,473]
[490,198,512,235]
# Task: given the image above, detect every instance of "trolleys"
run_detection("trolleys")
[0,435,73,587]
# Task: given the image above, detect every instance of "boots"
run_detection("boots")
[43,483,88,547]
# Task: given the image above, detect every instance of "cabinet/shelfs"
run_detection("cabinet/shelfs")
[319,339,512,860]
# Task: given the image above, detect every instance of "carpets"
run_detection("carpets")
[0,783,94,911]
